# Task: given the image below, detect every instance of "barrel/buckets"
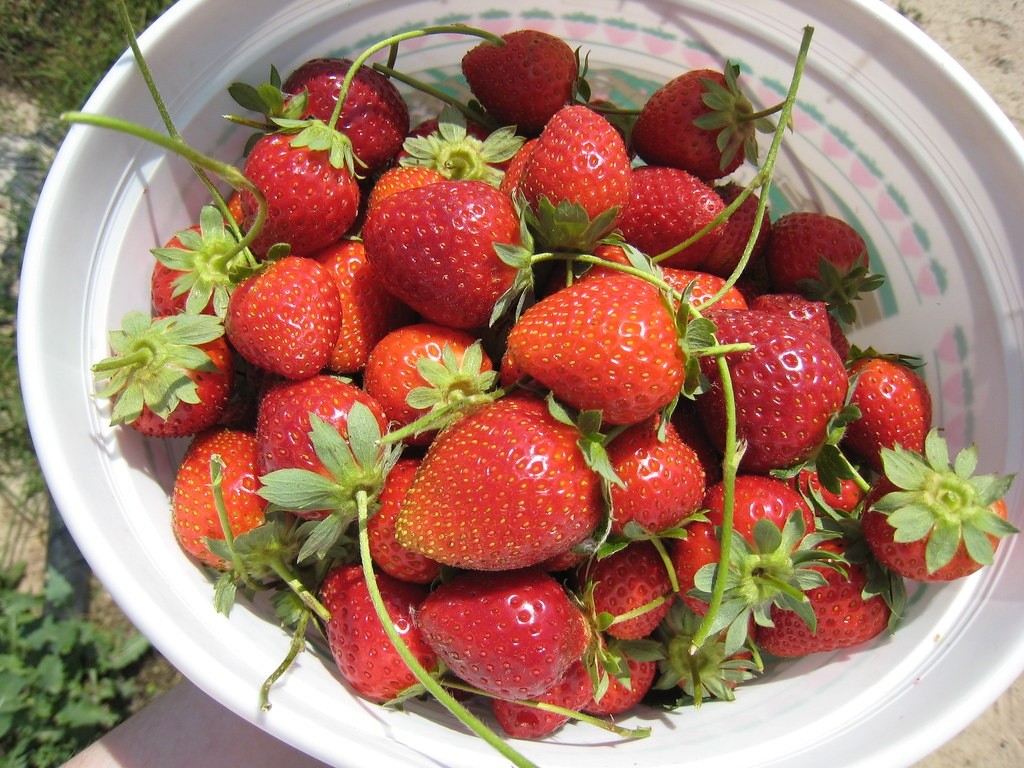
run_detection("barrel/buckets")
[18,1,1023,768]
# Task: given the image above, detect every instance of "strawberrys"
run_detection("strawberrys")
[88,21,1020,768]
